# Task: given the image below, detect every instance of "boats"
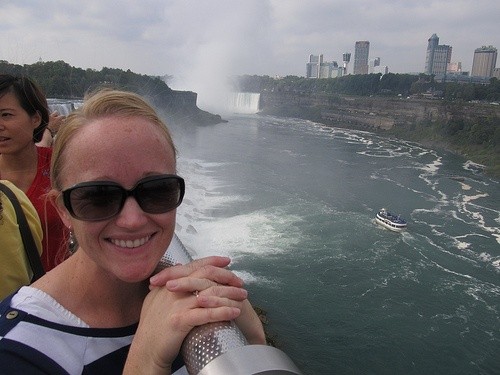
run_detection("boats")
[374,207,409,232]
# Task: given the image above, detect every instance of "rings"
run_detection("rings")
[194,290,203,307]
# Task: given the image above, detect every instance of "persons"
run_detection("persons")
[0,180,43,302]
[0,74,78,282]
[0,89,266,375]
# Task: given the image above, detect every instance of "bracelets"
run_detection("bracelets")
[46,126,53,136]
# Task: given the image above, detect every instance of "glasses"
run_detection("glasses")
[62,174,187,222]
